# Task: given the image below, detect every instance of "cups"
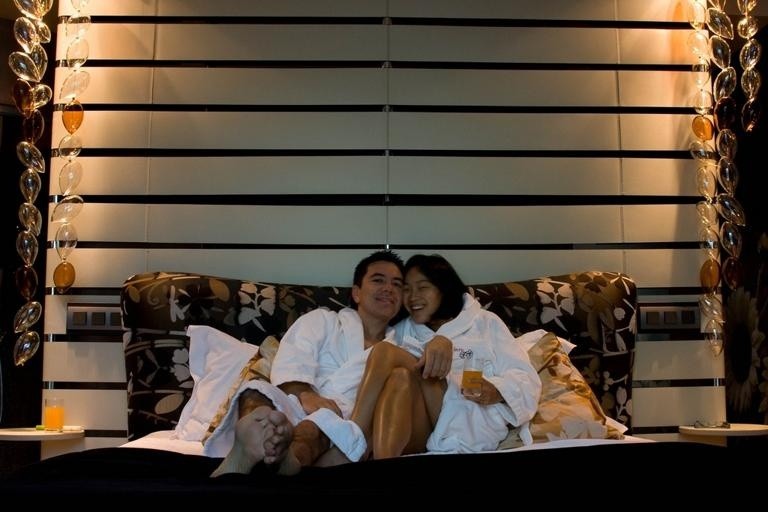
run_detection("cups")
[44,398,64,431]
[461,357,484,394]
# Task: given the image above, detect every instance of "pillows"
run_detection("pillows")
[176,322,279,445]
[491,330,627,446]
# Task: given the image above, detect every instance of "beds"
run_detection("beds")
[2,271,768,510]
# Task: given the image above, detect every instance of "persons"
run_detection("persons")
[312,253,542,467]
[208,251,454,476]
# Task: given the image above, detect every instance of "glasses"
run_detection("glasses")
[694,420,729,428]
